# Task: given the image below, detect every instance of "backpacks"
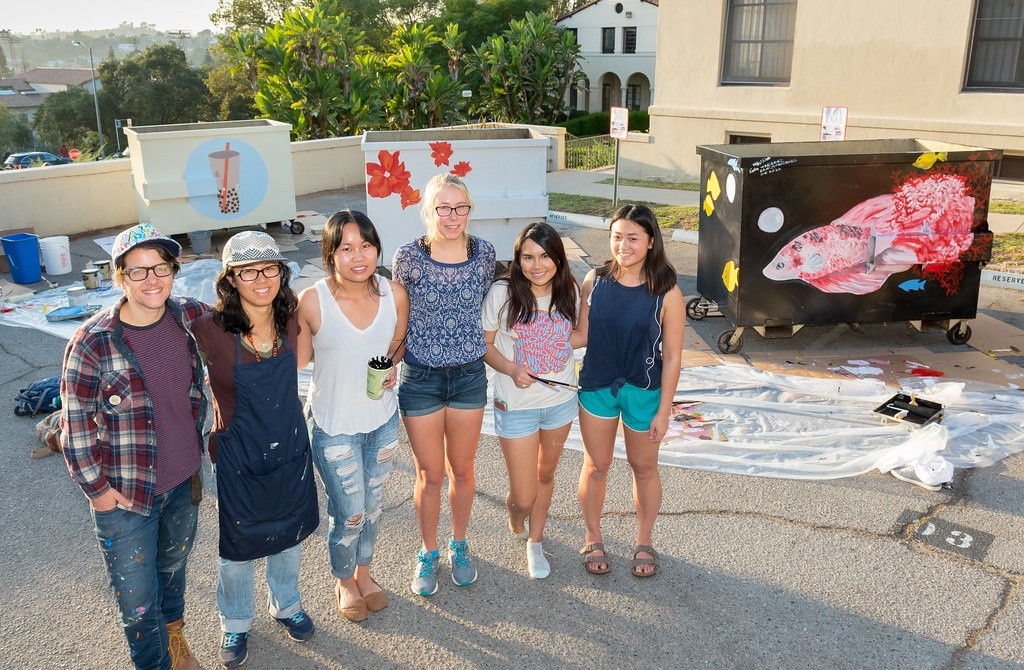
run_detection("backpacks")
[14,375,63,420]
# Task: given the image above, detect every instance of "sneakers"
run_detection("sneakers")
[410,547,441,596]
[447,535,478,586]
[219,629,252,669]
[166,618,200,670]
[269,609,316,642]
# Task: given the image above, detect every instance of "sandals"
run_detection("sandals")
[579,543,611,576]
[630,544,660,578]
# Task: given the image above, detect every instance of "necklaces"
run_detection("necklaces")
[251,328,271,349]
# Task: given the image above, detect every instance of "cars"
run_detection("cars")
[3,151,73,170]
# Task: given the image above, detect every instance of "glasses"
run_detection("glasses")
[232,263,284,282]
[433,205,472,217]
[120,261,175,281]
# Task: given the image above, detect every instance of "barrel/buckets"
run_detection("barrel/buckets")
[0,232,41,284]
[81,269,102,289]
[187,230,212,255]
[67,286,88,307]
[92,260,112,281]
[39,235,73,275]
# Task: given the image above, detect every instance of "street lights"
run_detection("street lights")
[72,39,105,160]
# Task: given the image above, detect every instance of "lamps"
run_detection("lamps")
[626,12,631,18]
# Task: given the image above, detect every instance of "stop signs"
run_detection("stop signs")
[69,149,81,160]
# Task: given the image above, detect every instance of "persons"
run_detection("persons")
[35,224,215,670]
[59,145,69,158]
[88,142,99,161]
[3,149,11,160]
[390,173,497,596]
[188,230,320,670]
[567,203,686,577]
[293,209,409,620]
[480,222,581,579]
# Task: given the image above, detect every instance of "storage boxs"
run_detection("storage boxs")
[358,123,551,269]
[687,138,1005,353]
[0,227,34,272]
[124,116,305,236]
[45,303,101,322]
[872,393,946,431]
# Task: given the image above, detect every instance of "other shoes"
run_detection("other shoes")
[355,575,388,613]
[335,582,368,622]
[889,463,943,492]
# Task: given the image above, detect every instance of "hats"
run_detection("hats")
[222,231,289,267]
[112,223,183,269]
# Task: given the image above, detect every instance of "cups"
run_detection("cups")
[366,356,394,400]
